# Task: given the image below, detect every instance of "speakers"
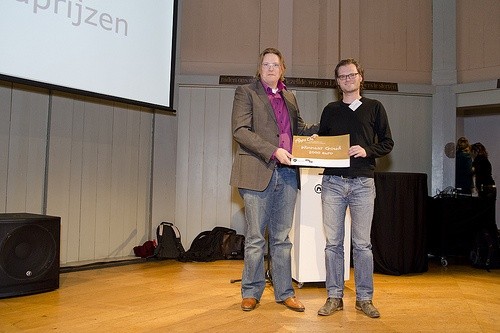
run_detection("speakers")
[0,212,61,299]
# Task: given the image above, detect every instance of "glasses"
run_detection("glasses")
[337,73,359,80]
[260,63,281,69]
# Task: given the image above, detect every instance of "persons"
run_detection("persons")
[230,48,320,312]
[311,59,394,318]
[455,136,500,244]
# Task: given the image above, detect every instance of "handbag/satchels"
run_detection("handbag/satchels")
[222,235,245,260]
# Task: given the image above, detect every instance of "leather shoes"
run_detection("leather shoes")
[242,298,256,311]
[277,296,305,312]
[355,300,380,318]
[318,298,343,316]
